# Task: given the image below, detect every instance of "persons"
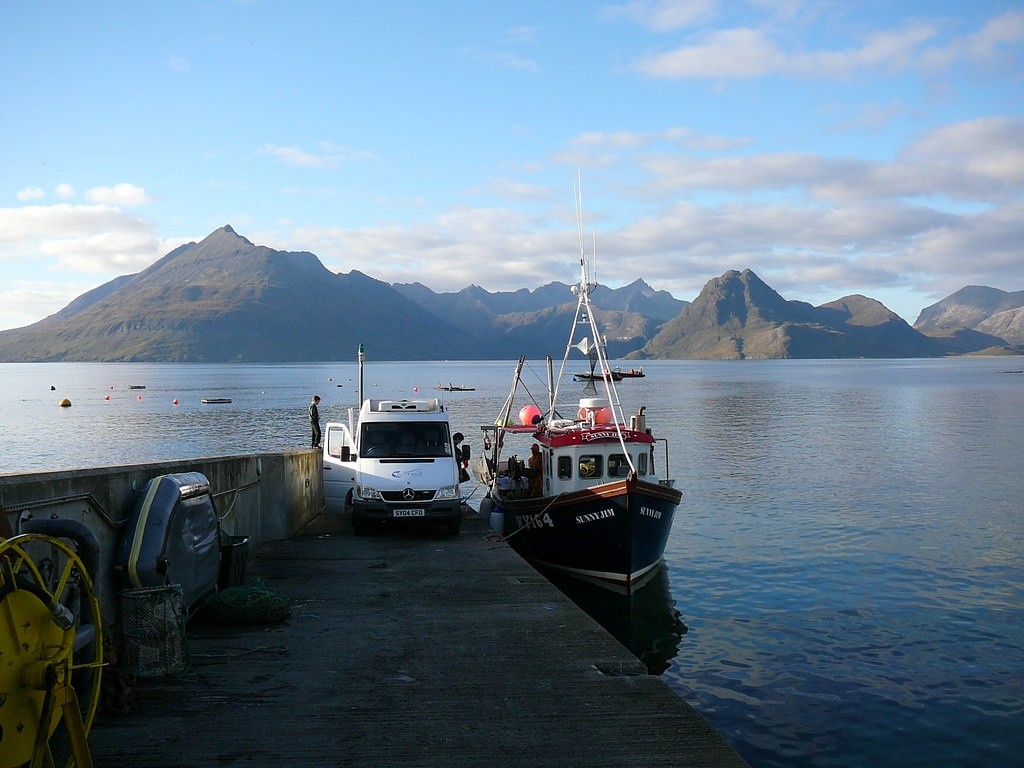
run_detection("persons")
[528,444,542,480]
[308,395,322,449]
[453,432,468,469]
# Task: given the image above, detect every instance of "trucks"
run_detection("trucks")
[322,399,471,537]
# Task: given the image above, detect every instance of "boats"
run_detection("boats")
[480,168,684,585]
[438,382,475,392]
[614,367,645,378]
[129,384,147,390]
[201,397,232,403]
[574,371,623,381]
